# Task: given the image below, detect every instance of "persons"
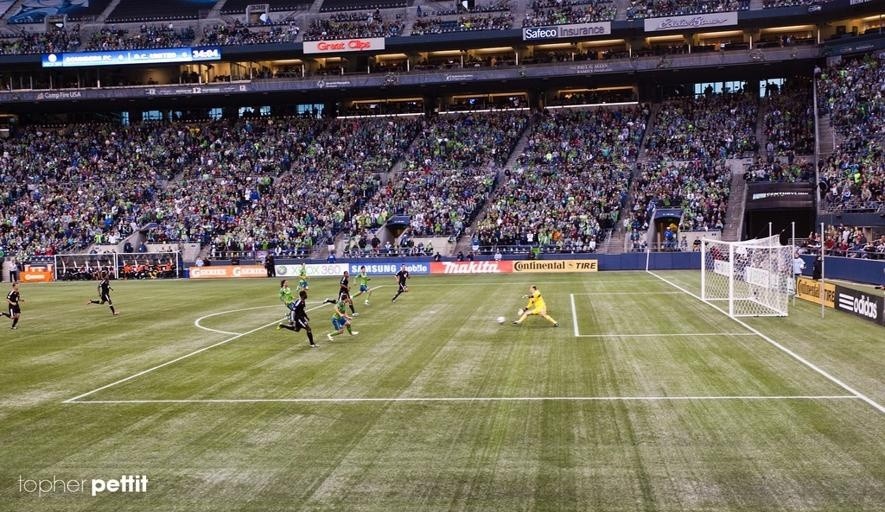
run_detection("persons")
[0,0,885,348]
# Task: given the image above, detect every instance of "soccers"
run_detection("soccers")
[496,316,505,324]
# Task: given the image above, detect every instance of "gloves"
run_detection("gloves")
[517,307,523,315]
[522,295,529,298]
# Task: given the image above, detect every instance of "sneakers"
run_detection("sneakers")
[364,299,369,304]
[350,331,357,335]
[327,333,333,341]
[554,323,558,327]
[352,312,358,316]
[391,298,396,302]
[513,320,519,324]
[322,298,329,303]
[276,323,281,330]
[310,343,319,348]
